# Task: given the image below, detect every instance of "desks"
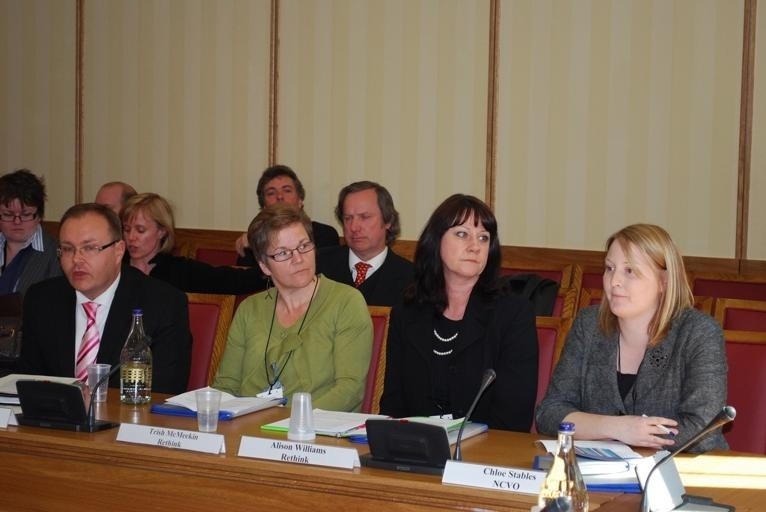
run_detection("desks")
[0,381,766,511]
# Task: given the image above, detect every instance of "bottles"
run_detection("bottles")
[120,309,153,405]
[538,421,588,512]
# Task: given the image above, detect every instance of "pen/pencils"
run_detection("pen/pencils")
[640,411,674,436]
[357,416,394,430]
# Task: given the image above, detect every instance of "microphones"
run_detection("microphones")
[540,497,571,512]
[640,406,736,512]
[453,368,497,461]
[86,335,154,432]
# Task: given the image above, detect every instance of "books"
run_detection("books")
[350,423,489,444]
[532,435,651,492]
[165,385,273,417]
[263,408,447,433]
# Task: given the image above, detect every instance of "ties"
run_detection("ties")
[354,262,372,287]
[76,302,101,388]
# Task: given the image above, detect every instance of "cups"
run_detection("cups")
[194,388,222,434]
[286,391,315,442]
[85,363,111,403]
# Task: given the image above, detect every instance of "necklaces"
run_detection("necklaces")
[263,282,317,393]
[432,327,459,358]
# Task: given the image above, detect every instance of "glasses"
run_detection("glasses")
[55,239,118,258]
[0,208,40,221]
[263,237,317,262]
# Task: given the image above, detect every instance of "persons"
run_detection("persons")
[234,162,340,271]
[90,181,138,221]
[21,202,194,398]
[1,167,66,365]
[116,191,215,291]
[532,222,731,452]
[381,192,541,432]
[314,178,425,306]
[213,202,374,412]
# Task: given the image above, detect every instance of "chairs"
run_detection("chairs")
[186,291,235,394]
[495,257,766,453]
[362,304,393,417]
[169,231,252,270]
